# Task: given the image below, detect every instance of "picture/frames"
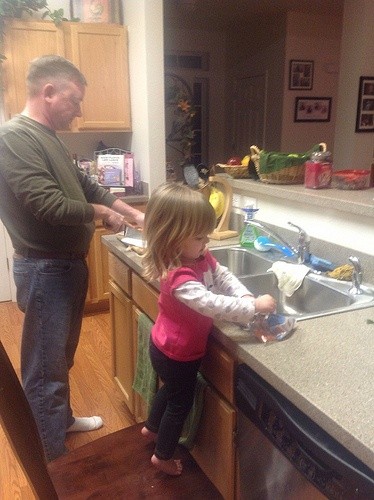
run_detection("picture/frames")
[287,59,315,89]
[356,75,374,134]
[295,96,332,123]
[69,0,124,24]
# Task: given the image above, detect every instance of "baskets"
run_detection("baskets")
[216,161,255,179]
[250,142,327,184]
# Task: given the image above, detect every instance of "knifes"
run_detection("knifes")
[102,221,147,248]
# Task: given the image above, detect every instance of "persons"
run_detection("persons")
[141,181,277,476]
[0,54,144,461]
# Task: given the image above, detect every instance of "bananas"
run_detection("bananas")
[209,186,225,219]
[242,155,251,165]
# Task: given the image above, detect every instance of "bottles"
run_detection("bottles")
[305,151,332,189]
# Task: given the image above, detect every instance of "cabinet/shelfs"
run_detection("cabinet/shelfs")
[106,252,135,419]
[76,203,152,308]
[0,16,133,133]
[130,270,238,500]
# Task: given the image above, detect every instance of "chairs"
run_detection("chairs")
[0,341,225,500]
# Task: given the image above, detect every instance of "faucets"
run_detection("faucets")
[243,220,311,264]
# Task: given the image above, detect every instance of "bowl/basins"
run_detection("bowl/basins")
[332,169,371,190]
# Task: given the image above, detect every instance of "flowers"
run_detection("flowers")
[167,85,198,160]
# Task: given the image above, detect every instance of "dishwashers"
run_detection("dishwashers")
[234,362,374,500]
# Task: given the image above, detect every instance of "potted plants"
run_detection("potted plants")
[0,0,80,28]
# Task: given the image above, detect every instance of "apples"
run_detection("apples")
[227,157,241,165]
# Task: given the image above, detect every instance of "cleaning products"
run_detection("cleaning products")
[240,208,260,249]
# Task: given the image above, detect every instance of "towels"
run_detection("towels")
[178,369,209,451]
[131,313,158,416]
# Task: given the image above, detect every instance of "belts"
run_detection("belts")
[16,247,86,260]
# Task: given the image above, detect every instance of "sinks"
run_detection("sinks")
[207,243,273,278]
[235,273,374,331]
